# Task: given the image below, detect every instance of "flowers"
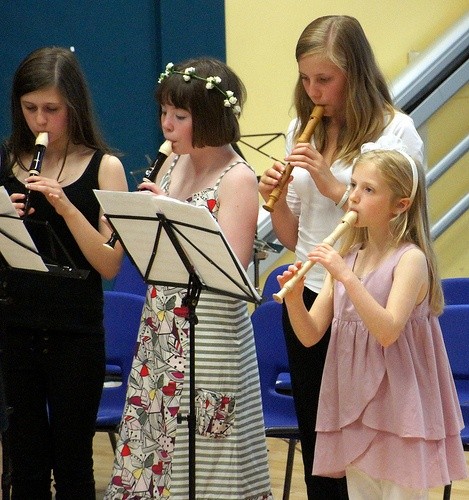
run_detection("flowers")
[158,62,241,114]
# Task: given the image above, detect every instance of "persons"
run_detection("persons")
[102,55,273,500]
[0,45,130,500]
[276,141,469,500]
[257,15,426,500]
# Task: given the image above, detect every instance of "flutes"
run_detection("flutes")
[20,130,51,226]
[273,210,358,305]
[262,104,325,213]
[101,139,174,253]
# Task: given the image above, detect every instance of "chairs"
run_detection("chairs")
[94,255,469,500]
